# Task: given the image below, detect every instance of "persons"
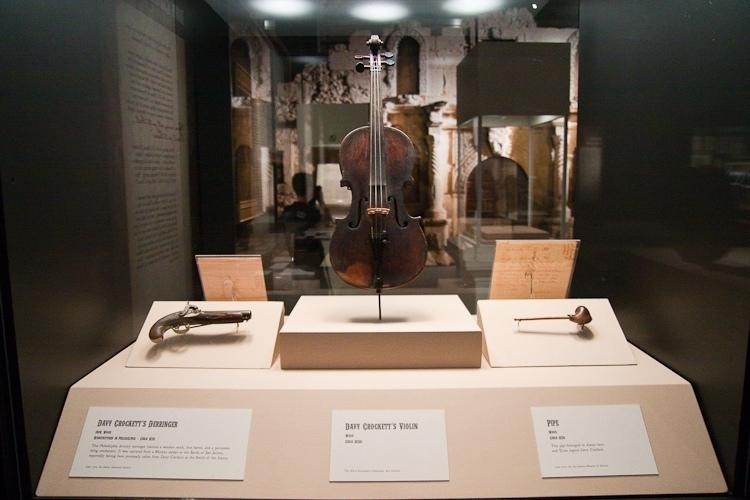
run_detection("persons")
[271,172,333,269]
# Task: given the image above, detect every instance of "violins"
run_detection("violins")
[329,34,429,292]
